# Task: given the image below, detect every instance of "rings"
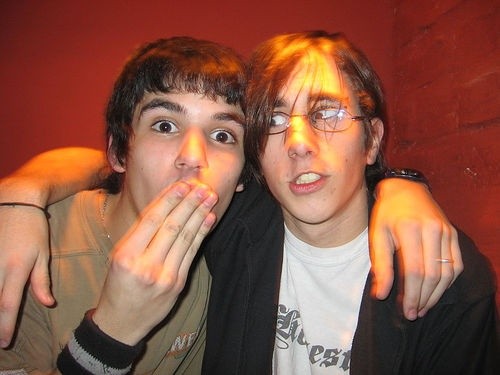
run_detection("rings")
[440,258,454,264]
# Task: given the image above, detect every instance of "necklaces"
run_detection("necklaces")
[98,191,113,241]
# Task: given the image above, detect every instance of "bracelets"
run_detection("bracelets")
[0,202,50,219]
[368,168,432,196]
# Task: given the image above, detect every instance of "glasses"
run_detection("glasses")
[268,106,369,135]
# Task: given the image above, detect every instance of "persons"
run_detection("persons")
[0,35,464,375]
[0,30,499,375]
